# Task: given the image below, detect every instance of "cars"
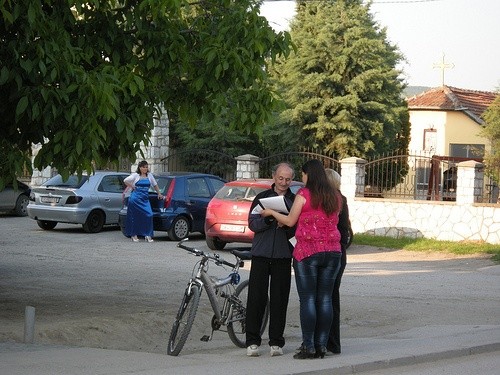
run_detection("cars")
[117,171,232,241]
[0,169,32,217]
[25,169,132,234]
[204,178,353,251]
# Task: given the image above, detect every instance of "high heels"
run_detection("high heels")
[131,236,139,242]
[145,235,154,243]
[316,347,327,359]
[293,350,315,359]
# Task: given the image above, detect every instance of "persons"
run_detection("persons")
[246,163,298,356]
[260,160,342,359]
[123,160,164,242]
[324,168,349,356]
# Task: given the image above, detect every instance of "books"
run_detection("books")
[259,196,291,215]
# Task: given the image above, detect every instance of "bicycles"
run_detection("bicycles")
[166,238,268,357]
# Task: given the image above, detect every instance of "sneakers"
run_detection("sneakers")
[246,345,259,356]
[270,346,283,356]
